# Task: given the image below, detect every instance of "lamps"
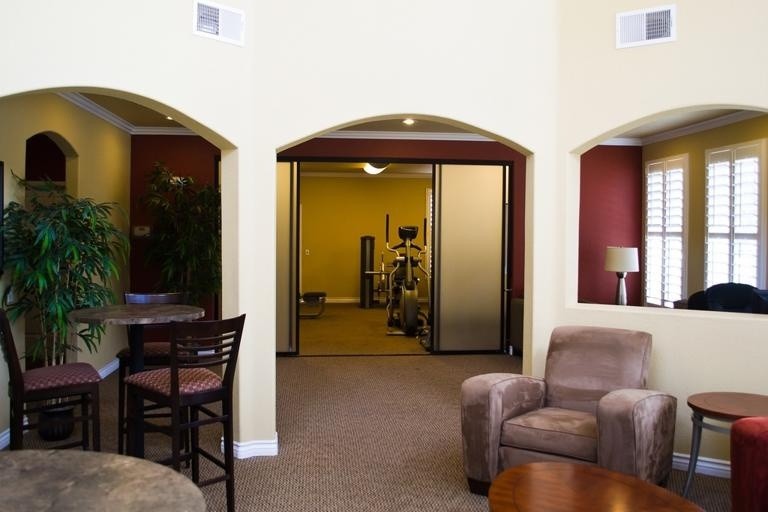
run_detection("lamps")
[604,246,640,305]
[362,161,392,175]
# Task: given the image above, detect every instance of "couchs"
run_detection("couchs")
[687,283,767,313]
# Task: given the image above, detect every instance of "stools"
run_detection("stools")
[299,292,326,316]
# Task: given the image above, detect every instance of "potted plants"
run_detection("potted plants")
[0,167,131,441]
[138,158,221,365]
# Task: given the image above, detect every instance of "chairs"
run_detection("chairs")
[116,291,192,467]
[0,307,101,450]
[461,326,678,496]
[732,418,768,510]
[122,313,245,511]
[395,253,419,285]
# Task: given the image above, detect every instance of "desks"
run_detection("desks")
[0,448,205,511]
[489,461,710,512]
[66,303,206,459]
[682,392,768,495]
